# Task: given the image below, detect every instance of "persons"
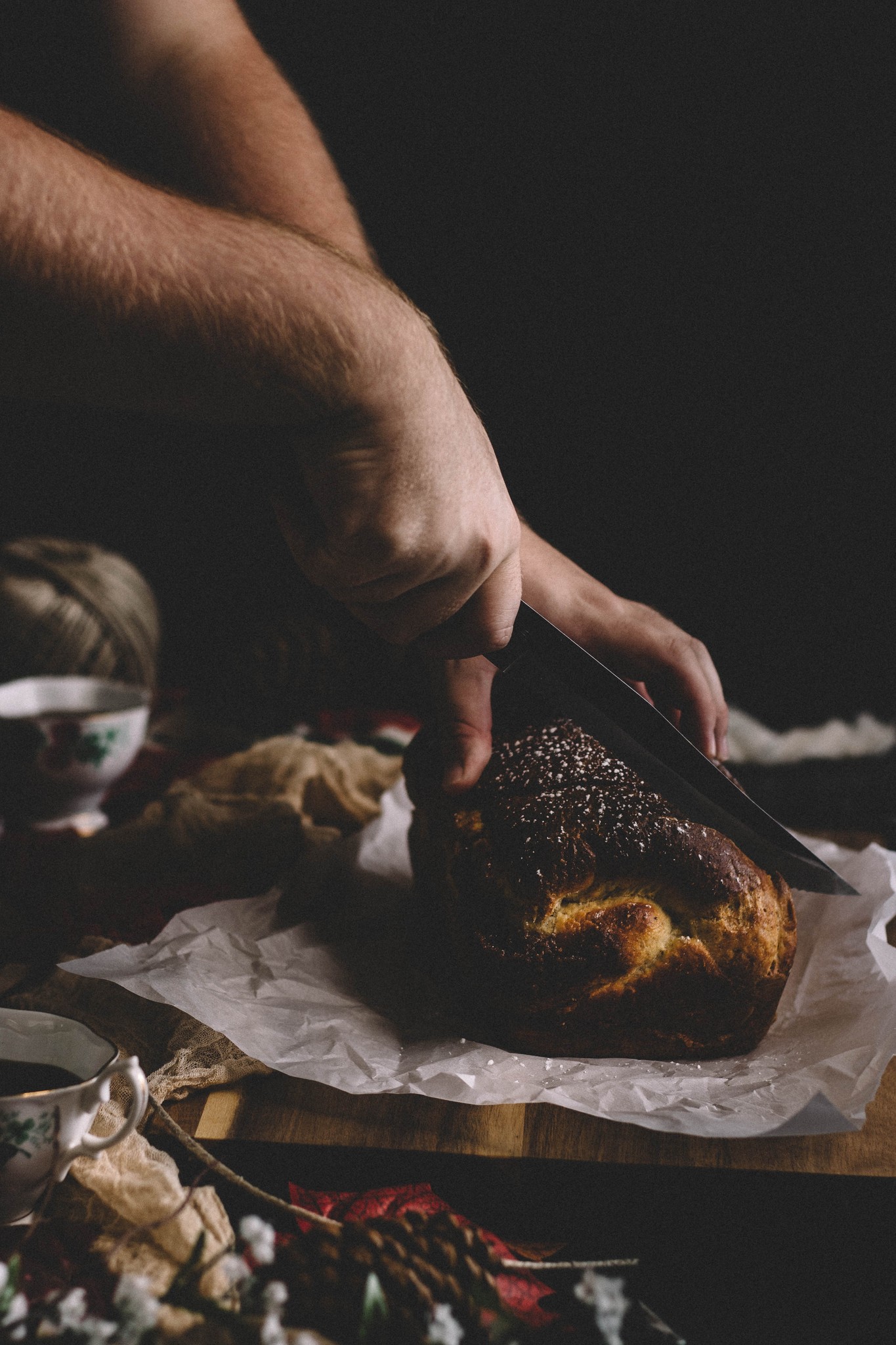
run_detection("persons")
[3,2,730,803]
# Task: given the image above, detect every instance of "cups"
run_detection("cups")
[0,1002,148,1227]
[1,670,156,834]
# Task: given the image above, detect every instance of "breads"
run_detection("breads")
[328,718,797,1062]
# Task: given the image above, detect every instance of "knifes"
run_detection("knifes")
[464,579,867,909]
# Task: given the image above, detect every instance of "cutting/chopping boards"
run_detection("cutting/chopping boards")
[137,855,894,1199]
